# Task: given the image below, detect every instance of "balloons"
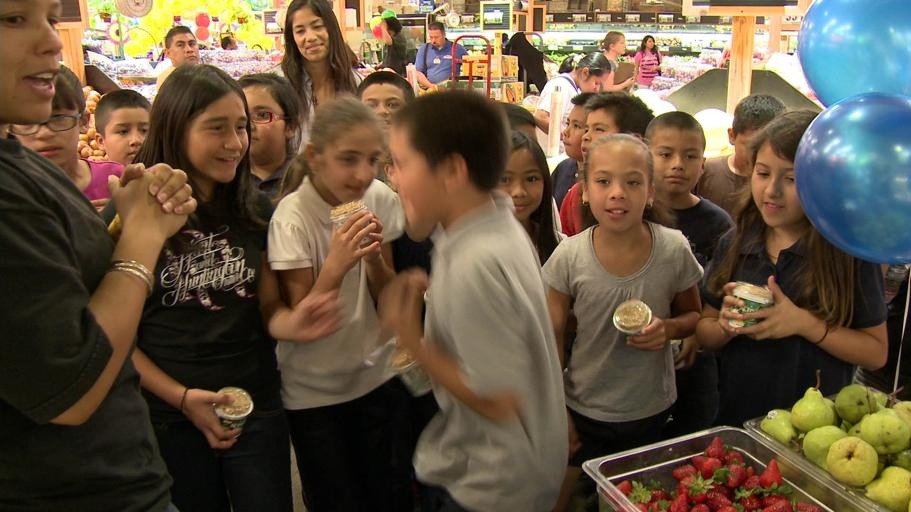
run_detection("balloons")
[797,0,911,109]
[793,90,911,263]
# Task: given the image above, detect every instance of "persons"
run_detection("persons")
[94,89,152,167]
[549,91,599,212]
[533,51,611,159]
[693,108,890,430]
[266,94,408,512]
[234,73,295,200]
[493,131,569,266]
[264,0,365,154]
[356,72,432,277]
[375,17,406,76]
[7,64,124,215]
[599,31,634,94]
[540,133,704,512]
[415,22,469,90]
[0,0,197,512]
[559,91,654,236]
[645,111,737,429]
[377,91,570,512]
[691,93,786,226]
[502,33,510,47]
[631,35,662,89]
[98,59,348,512]
[155,26,199,92]
[221,36,238,50]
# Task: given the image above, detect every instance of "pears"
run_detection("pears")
[873,386,904,407]
[791,370,833,431]
[844,466,911,511]
[892,451,910,470]
[761,409,800,449]
[827,435,878,486]
[835,384,875,424]
[841,420,853,432]
[802,426,846,470]
[849,423,861,435]
[860,409,909,453]
[894,401,911,428]
[822,397,837,423]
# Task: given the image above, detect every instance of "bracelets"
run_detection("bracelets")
[105,259,155,297]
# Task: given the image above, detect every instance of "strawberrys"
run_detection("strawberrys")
[670,435,781,491]
[613,478,821,512]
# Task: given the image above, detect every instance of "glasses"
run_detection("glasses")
[7,113,83,136]
[244,111,288,123]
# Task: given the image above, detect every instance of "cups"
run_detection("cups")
[612,299,653,336]
[726,280,772,331]
[671,337,685,370]
[328,198,374,247]
[214,406,253,432]
[391,360,432,395]
